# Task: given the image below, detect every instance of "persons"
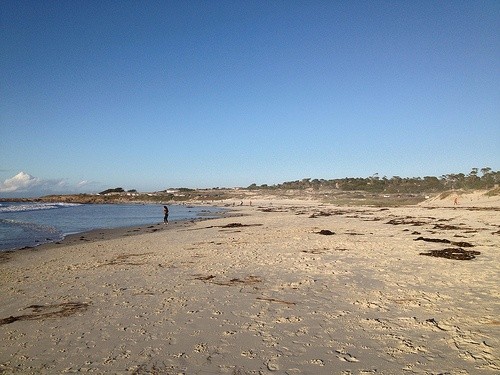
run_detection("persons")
[163,205,170,225]
[454,197,458,205]
